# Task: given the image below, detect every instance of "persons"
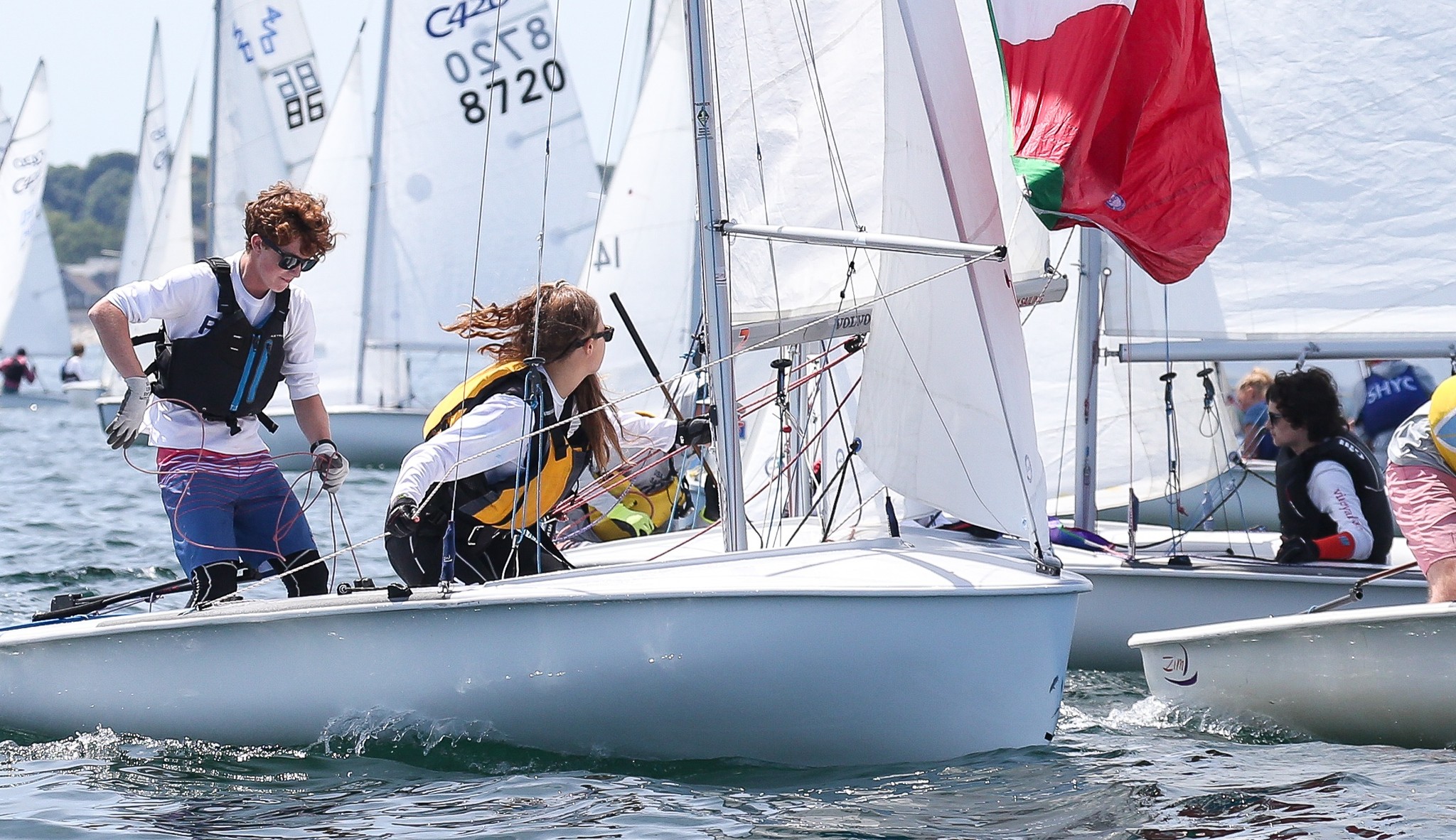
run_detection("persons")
[0,348,36,394]
[1235,358,1456,605]
[61,342,86,383]
[88,179,350,611]
[383,280,745,586]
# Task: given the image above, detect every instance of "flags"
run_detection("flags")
[985,0,1233,286]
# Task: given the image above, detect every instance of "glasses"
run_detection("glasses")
[260,238,320,272]
[1268,411,1285,426]
[578,325,614,348]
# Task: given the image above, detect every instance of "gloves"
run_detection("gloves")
[105,376,151,450]
[606,502,659,538]
[312,438,350,494]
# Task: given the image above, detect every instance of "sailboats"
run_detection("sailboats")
[1045,1,1456,748]
[0,1,1093,774]
